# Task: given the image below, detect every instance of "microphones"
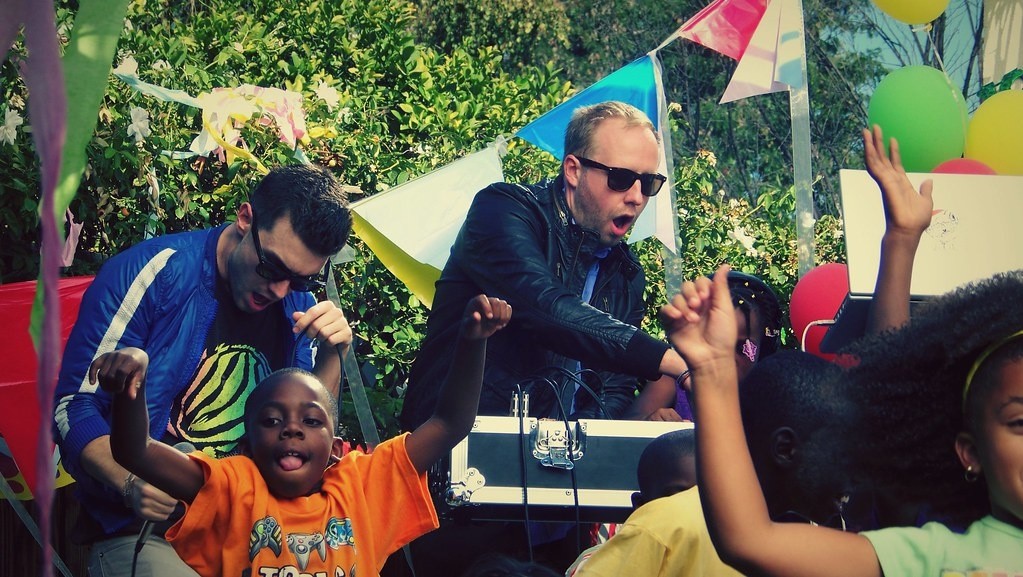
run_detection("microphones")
[137,441,196,552]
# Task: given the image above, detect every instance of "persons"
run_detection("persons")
[564,124,1023,577]
[50,164,512,577]
[402,101,689,577]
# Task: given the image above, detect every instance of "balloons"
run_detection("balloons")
[868,0,1023,175]
[790,263,861,367]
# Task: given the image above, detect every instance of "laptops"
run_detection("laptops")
[818,168,1023,365]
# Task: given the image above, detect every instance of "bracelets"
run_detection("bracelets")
[677,369,690,384]
[120,472,135,496]
[642,409,657,421]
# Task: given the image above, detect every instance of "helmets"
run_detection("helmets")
[706,271,784,364]
[789,263,850,364]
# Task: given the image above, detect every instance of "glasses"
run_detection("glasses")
[251,209,331,293]
[576,157,667,197]
